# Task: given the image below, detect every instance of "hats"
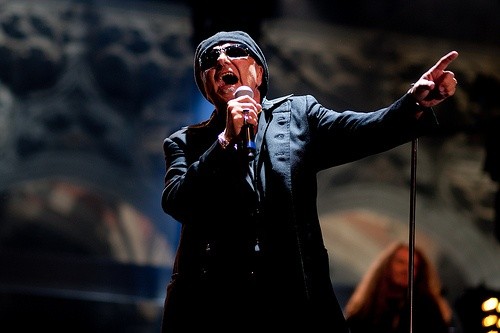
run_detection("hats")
[194,31,269,107]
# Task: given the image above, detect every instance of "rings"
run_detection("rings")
[241,109,249,124]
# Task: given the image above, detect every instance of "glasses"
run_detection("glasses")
[200,45,250,67]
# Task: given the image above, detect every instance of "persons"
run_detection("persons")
[161,28,459,332]
[339,238,458,332]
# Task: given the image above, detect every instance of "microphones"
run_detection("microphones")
[234,85,256,160]
